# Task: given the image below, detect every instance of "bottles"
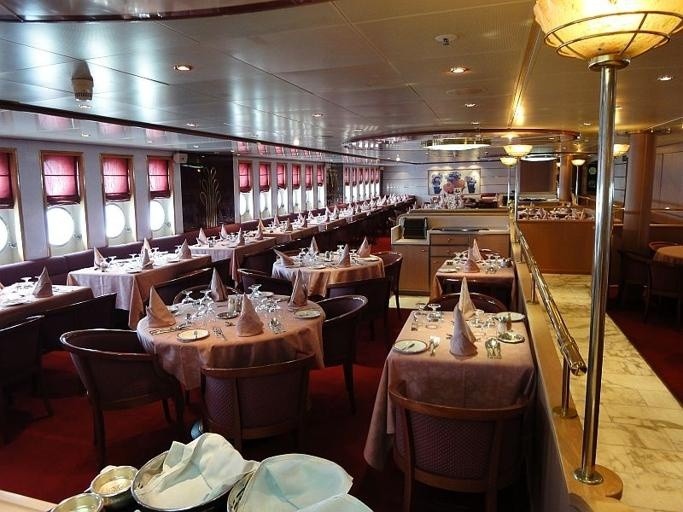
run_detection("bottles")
[501,257,512,267]
[330,252,334,261]
[498,312,512,333]
[325,251,329,261]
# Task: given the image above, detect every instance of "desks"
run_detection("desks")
[653,245,683,266]
[0,194,535,512]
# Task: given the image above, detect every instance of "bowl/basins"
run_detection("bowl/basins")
[89,464,138,511]
[50,492,106,512]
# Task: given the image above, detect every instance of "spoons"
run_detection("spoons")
[488,338,499,356]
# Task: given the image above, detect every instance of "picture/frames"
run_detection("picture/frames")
[427,168,482,194]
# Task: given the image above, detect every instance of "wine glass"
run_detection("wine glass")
[252,284,263,299]
[200,290,214,306]
[486,254,493,266]
[299,246,317,260]
[494,256,501,270]
[182,290,194,305]
[337,243,358,263]
[193,231,273,247]
[427,304,441,320]
[100,244,186,272]
[249,287,260,302]
[414,304,426,321]
[266,220,309,231]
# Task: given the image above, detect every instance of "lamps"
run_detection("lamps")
[612,143,629,157]
[570,159,586,202]
[499,157,517,205]
[503,144,533,218]
[532,0,683,501]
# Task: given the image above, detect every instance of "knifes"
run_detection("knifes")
[497,342,502,359]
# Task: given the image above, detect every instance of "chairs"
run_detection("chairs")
[649,241,679,257]
[616,246,651,309]
[647,258,680,326]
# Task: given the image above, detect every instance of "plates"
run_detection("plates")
[309,264,325,268]
[2,297,27,305]
[438,268,456,273]
[496,312,525,321]
[394,339,427,354]
[166,292,321,341]
[497,332,524,343]
